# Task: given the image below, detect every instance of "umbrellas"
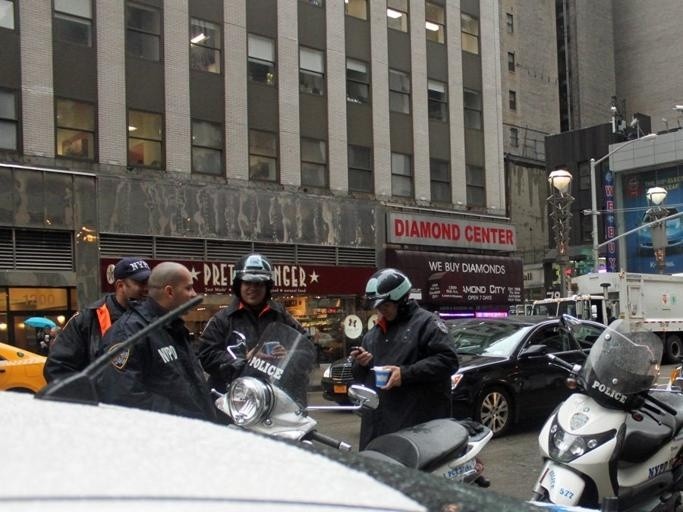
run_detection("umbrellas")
[24,317,57,333]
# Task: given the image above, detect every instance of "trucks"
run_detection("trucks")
[528,269,682,365]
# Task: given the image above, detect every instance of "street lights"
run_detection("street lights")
[589,133,658,271]
[546,167,576,297]
[644,186,669,275]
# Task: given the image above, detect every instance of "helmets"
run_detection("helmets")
[235,254,274,282]
[364,268,412,310]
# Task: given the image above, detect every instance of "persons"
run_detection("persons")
[42,255,152,406]
[338,267,459,460]
[196,254,315,432]
[37,325,62,355]
[93,261,222,428]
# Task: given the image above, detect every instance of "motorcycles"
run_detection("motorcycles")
[209,321,495,490]
[527,318,683,511]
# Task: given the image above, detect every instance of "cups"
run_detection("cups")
[264,341,281,355]
[370,367,392,389]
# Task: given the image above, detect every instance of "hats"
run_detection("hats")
[114,258,151,282]
